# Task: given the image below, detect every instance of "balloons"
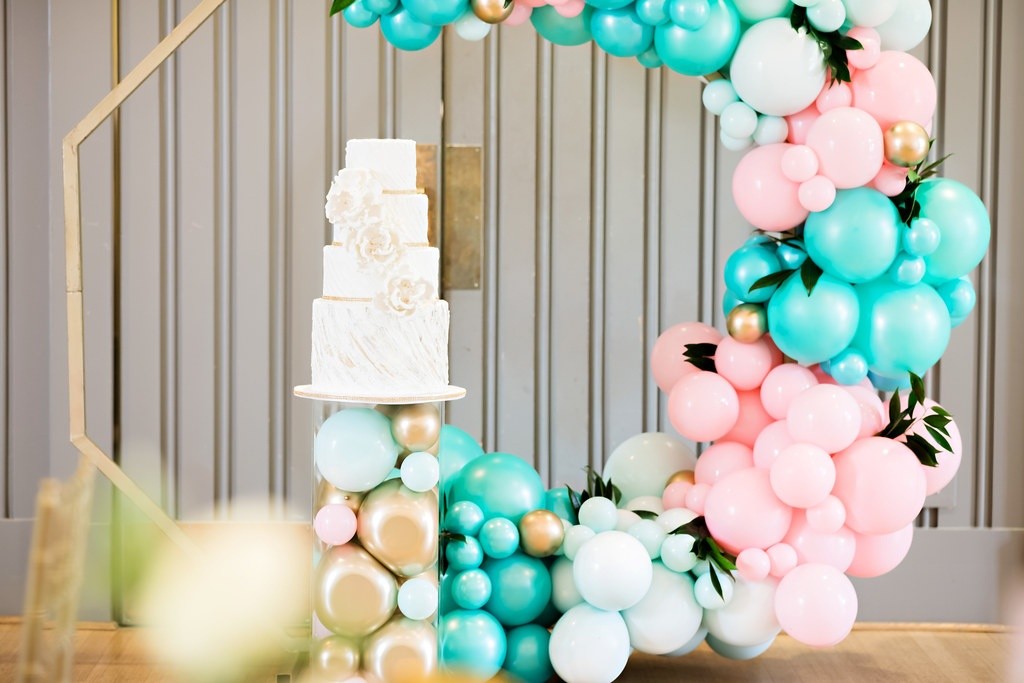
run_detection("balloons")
[314,1,994,683]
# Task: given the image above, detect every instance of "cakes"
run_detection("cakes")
[310,138,450,397]
